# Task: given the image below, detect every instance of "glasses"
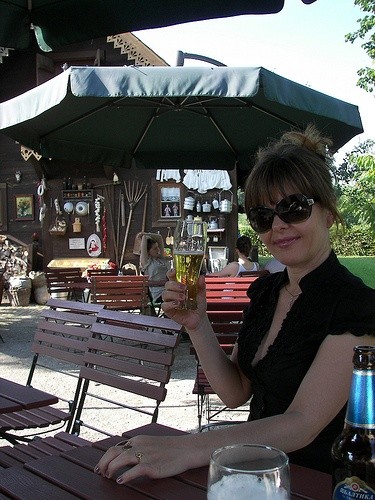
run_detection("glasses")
[247,193,319,234]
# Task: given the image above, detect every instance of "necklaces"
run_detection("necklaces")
[285,286,302,306]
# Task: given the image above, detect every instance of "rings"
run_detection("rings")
[121,442,132,451]
[135,450,144,463]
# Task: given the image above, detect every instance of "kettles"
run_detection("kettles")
[208,216,217,230]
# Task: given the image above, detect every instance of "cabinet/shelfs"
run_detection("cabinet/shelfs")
[62,189,94,202]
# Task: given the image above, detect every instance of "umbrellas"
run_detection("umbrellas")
[0,50,365,260]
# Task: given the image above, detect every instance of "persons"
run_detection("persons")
[205,236,259,299]
[136,232,172,302]
[93,124,375,485]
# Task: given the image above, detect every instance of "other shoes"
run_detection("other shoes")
[180,332,190,342]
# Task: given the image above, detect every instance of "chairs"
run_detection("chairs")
[0,268,272,473]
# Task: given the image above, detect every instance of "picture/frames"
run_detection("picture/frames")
[13,194,34,220]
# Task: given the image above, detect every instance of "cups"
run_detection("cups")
[172,219,207,311]
[206,443,292,500]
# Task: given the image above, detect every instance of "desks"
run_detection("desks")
[0,423,330,500]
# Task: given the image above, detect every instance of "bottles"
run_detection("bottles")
[331,345,375,500]
[184,189,234,213]
